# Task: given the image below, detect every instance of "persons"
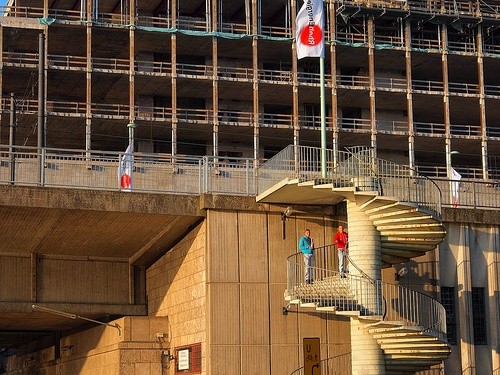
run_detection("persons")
[298,228,316,286]
[333,223,350,279]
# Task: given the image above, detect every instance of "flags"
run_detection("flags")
[117,142,133,192]
[451,167,463,209]
[294,0,326,59]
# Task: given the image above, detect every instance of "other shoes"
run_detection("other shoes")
[307,279,314,284]
[340,274,347,278]
